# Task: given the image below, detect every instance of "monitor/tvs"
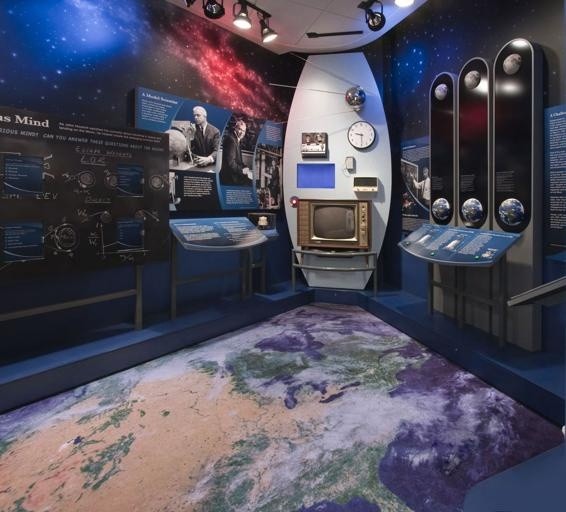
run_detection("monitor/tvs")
[297,163,335,188]
[297,198,372,252]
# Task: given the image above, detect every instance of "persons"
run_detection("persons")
[409,167,430,206]
[172,105,220,167]
[264,159,279,206]
[219,120,249,185]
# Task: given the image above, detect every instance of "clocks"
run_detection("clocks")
[348,121,376,149]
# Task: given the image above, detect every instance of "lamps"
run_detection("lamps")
[367,8,385,32]
[204,0,278,44]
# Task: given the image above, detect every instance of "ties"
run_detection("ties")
[201,126,204,138]
